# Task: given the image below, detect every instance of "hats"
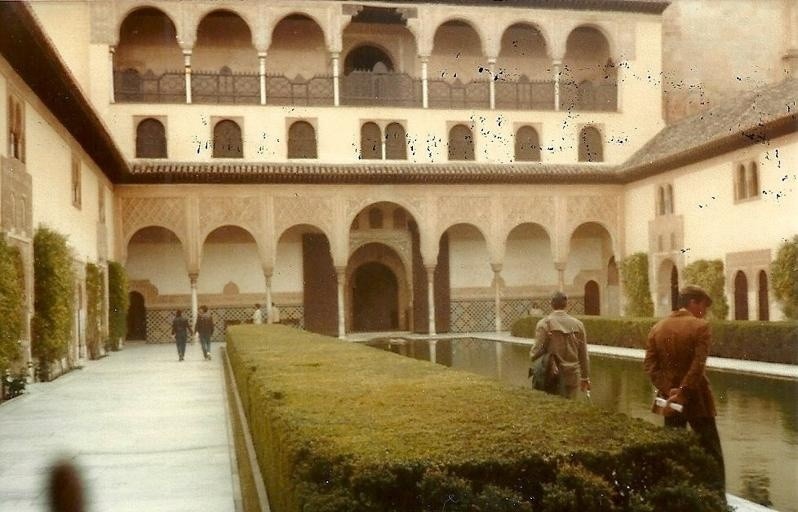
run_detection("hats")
[681,284,712,307]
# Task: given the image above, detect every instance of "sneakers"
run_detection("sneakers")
[178,352,213,361]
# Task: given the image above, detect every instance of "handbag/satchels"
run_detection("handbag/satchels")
[528,351,560,394]
[652,390,687,416]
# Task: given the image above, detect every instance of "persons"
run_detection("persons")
[193,305,214,360]
[643,285,725,500]
[272,302,280,324]
[531,290,592,400]
[172,311,193,361]
[251,303,262,324]
